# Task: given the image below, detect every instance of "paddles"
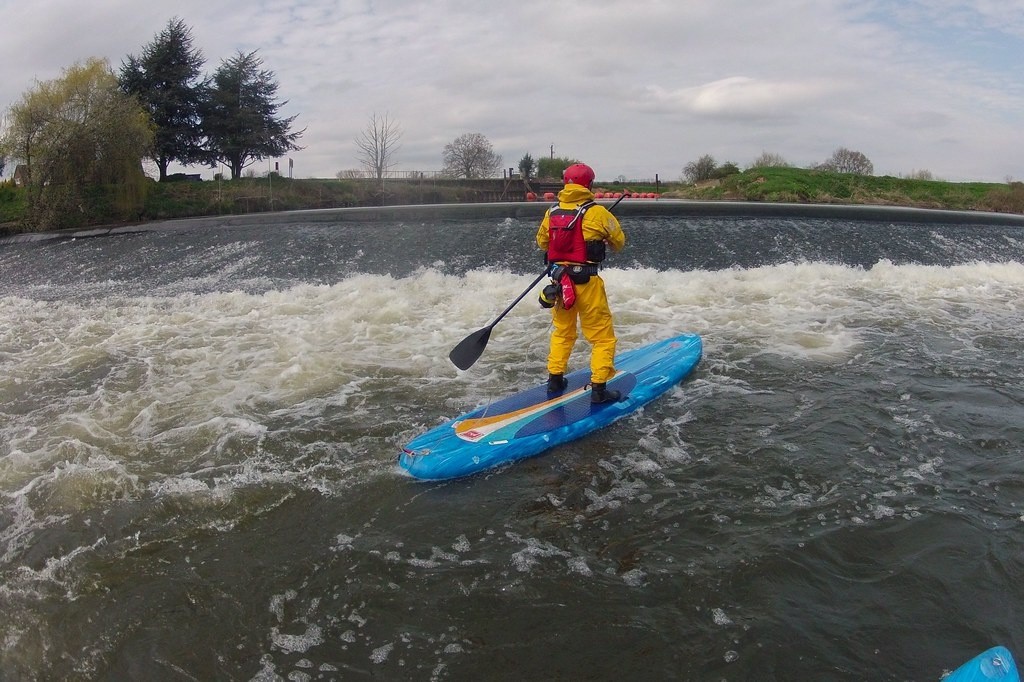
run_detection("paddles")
[448,193,627,371]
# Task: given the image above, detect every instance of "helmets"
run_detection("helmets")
[563,163,596,189]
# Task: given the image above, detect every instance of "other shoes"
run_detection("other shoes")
[547,373,568,391]
[590,382,621,403]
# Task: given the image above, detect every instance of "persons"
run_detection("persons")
[536,164,626,405]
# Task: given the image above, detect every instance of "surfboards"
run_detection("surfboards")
[940,644,1022,682]
[398,332,703,482]
